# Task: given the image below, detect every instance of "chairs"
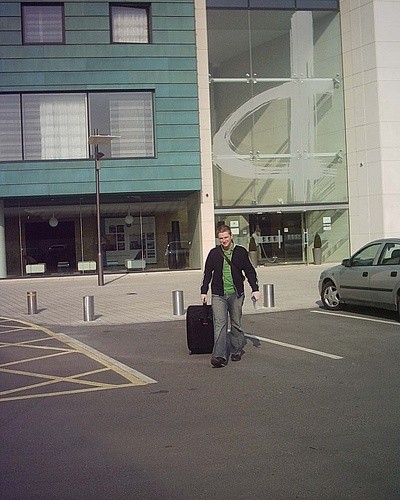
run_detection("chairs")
[383,250,400,264]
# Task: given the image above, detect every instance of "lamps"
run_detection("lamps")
[49,208,58,227]
[125,204,134,224]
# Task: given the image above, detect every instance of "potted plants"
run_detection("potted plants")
[313,233,322,265]
[248,237,257,268]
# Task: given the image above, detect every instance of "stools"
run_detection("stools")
[125,259,146,271]
[78,261,96,274]
[26,263,46,276]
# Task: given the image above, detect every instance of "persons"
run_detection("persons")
[200,225,261,367]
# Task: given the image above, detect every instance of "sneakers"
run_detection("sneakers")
[211,357,229,368]
[230,353,241,361]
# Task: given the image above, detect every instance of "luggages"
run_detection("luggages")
[186,301,214,355]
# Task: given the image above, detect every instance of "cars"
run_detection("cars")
[318,238,400,312]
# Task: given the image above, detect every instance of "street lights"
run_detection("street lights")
[88,135,121,286]
